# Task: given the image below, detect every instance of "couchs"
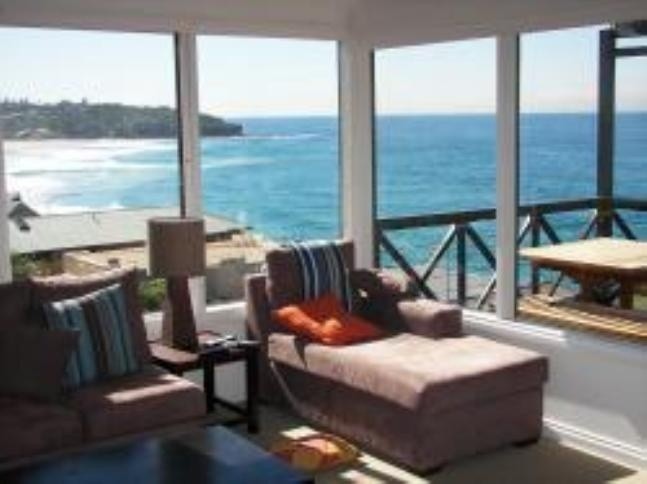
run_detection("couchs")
[0,259,218,469]
[241,238,551,477]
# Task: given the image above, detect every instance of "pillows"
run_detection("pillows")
[1,278,34,327]
[265,238,355,312]
[0,324,80,405]
[25,263,147,364]
[269,432,362,474]
[272,292,384,343]
[40,283,141,391]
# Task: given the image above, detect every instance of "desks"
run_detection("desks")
[517,235,647,311]
[147,329,262,436]
[0,424,316,484]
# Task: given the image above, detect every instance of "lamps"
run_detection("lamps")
[147,217,207,353]
